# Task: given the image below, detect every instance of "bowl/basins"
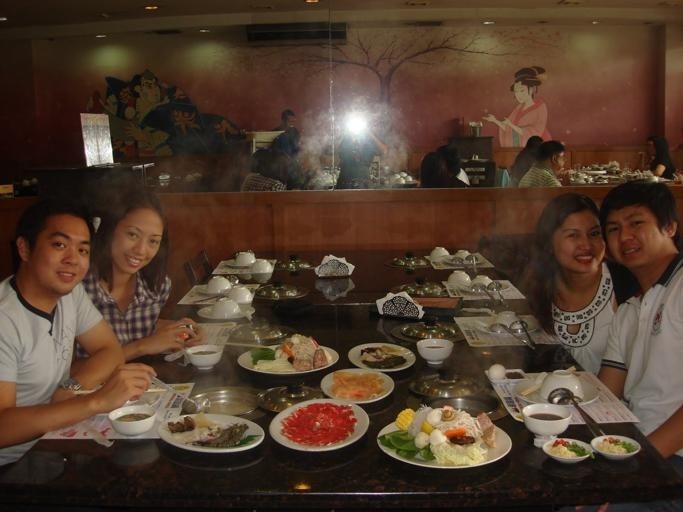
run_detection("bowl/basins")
[539,369,584,402]
[427,246,451,263]
[392,170,414,185]
[224,284,253,303]
[415,338,454,366]
[447,269,473,288]
[206,275,232,293]
[493,311,519,328]
[185,343,225,371]
[235,251,256,265]
[453,249,473,265]
[250,258,274,274]
[471,274,494,290]
[521,402,574,437]
[107,403,158,437]
[212,297,240,317]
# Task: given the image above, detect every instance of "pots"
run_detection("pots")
[180,385,266,418]
[390,320,465,344]
[273,255,313,273]
[385,256,430,270]
[420,386,510,422]
[392,280,450,298]
[227,316,291,345]
[254,281,310,300]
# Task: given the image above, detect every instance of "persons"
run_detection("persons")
[122,69,171,126]
[73,193,199,377]
[510,135,544,184]
[273,110,296,130]
[0,204,157,469]
[324,116,387,190]
[125,83,246,158]
[595,179,683,460]
[524,192,632,378]
[248,128,318,190]
[648,136,674,179]
[518,140,565,188]
[483,66,552,148]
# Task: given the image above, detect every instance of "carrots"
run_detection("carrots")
[445,428,466,436]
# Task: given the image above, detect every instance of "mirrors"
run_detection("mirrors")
[0,1,683,196]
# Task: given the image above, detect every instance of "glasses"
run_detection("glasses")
[557,154,567,164]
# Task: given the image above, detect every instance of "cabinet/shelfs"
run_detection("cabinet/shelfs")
[447,117,496,188]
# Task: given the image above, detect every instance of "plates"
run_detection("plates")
[114,391,162,412]
[157,412,267,457]
[224,259,255,268]
[347,342,417,371]
[483,368,530,384]
[590,433,642,462]
[423,253,486,266]
[236,342,340,377]
[508,379,602,407]
[541,437,592,465]
[444,279,513,294]
[196,304,256,320]
[319,367,395,406]
[473,319,539,337]
[196,286,229,296]
[268,398,370,454]
[376,418,514,470]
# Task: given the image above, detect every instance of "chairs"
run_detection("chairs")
[182,249,213,284]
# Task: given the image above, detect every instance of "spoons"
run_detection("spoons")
[485,280,514,313]
[147,374,213,414]
[471,307,499,312]
[465,253,483,279]
[548,386,605,438]
[489,322,538,353]
[509,321,539,350]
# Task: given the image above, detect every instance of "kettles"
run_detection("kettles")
[496,165,511,187]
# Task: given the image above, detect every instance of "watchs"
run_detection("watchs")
[59,376,83,392]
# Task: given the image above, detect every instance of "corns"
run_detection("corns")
[396,409,415,429]
